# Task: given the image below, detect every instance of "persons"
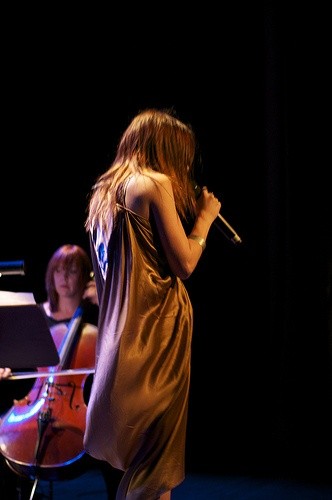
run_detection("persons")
[0,245,98,380]
[84,111,220,500]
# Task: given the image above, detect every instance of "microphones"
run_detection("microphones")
[190,183,241,245]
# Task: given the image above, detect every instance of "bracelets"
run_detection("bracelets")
[188,234,206,249]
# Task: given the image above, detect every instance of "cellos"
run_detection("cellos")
[1,271,99,500]
